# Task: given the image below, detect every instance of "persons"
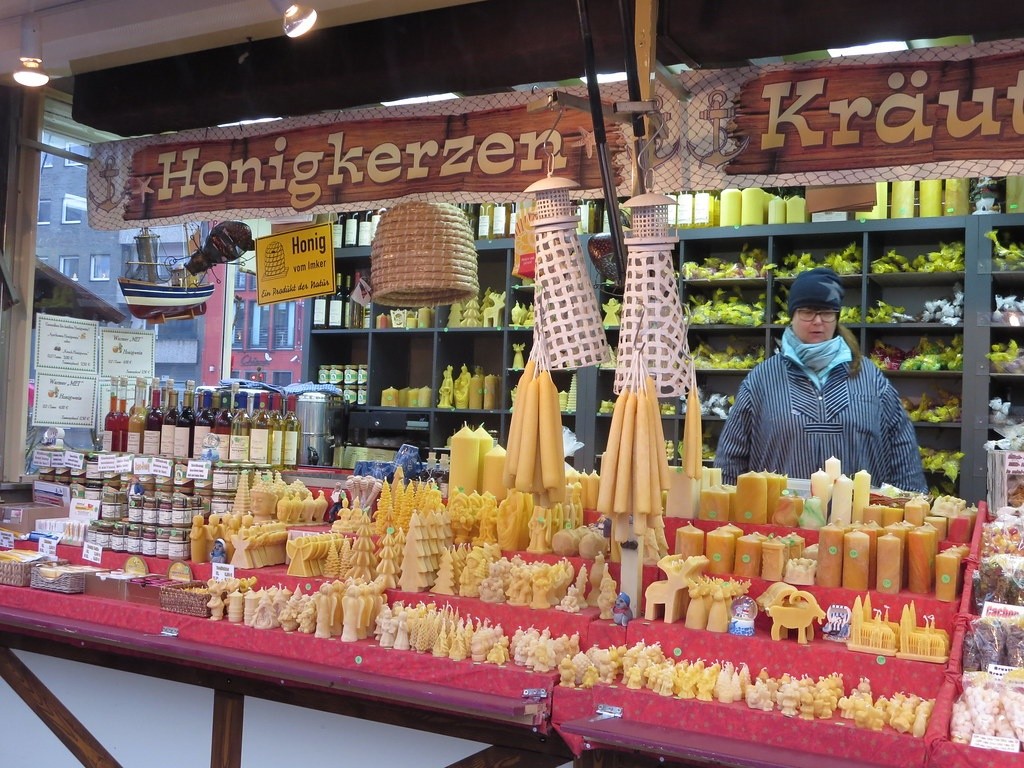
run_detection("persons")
[716,267,927,494]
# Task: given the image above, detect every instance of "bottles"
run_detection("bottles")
[453,199,516,240]
[104,375,301,472]
[664,190,720,229]
[569,199,611,235]
[332,208,388,249]
[312,272,371,330]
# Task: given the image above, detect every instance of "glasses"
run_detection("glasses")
[796,309,840,323]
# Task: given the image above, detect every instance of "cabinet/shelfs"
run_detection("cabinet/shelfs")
[974,210,1024,503]
[298,232,592,474]
[0,487,352,768]
[541,493,989,768]
[923,498,1024,768]
[0,484,695,768]
[580,215,978,509]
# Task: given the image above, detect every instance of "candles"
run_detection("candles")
[890,181,916,218]
[693,197,695,229]
[1005,175,1024,214]
[719,189,742,227]
[764,192,770,224]
[767,195,786,224]
[944,177,971,216]
[787,195,811,223]
[854,181,888,220]
[919,179,943,217]
[741,187,764,225]
[191,284,978,737]
[769,194,776,201]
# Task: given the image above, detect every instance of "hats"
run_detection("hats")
[787,267,847,316]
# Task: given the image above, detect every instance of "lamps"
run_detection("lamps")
[11,12,51,88]
[274,0,317,40]
[209,365,215,372]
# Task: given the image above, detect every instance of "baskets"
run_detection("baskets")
[371,201,479,307]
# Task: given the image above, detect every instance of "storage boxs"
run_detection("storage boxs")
[62,539,84,547]
[0,502,70,532]
[811,211,848,222]
[34,480,71,507]
[125,576,182,607]
[344,446,398,470]
[332,446,346,468]
[84,569,141,601]
[69,497,101,523]
